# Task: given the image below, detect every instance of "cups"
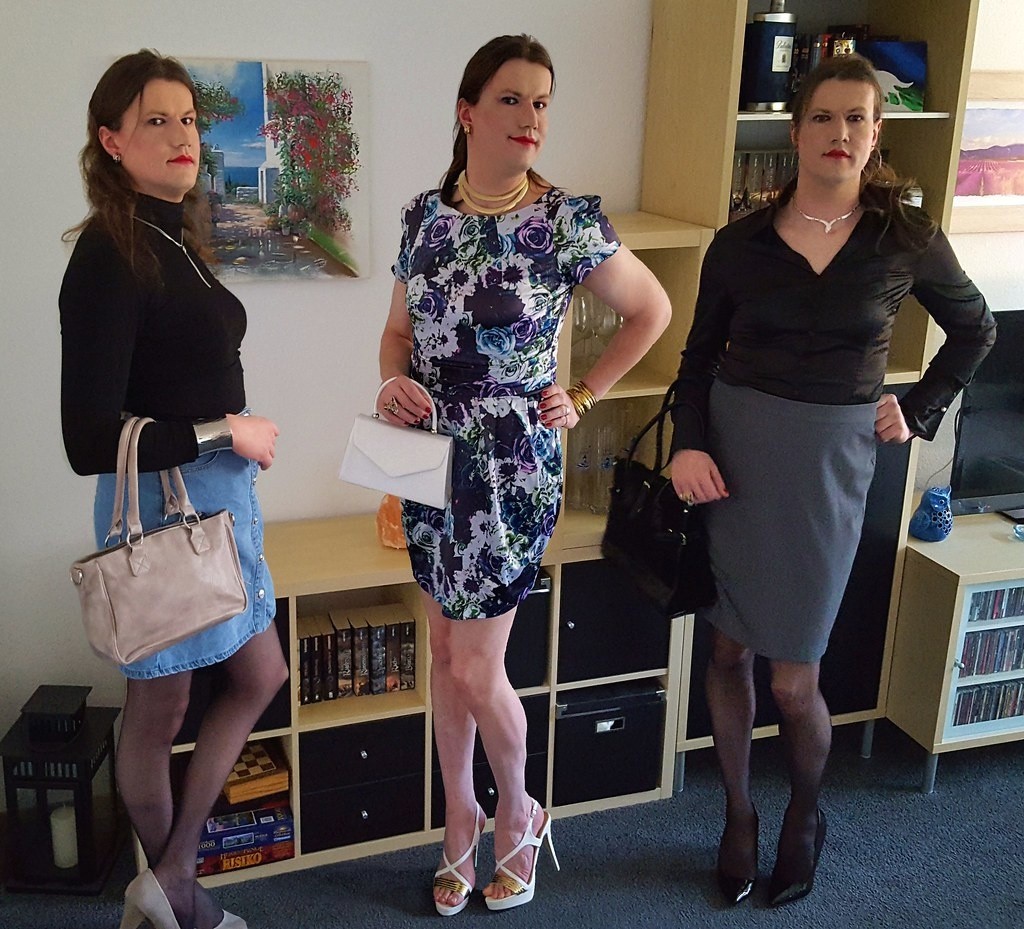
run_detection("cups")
[563,422,648,515]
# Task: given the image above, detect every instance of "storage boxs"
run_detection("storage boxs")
[552,679,667,807]
[504,569,551,690]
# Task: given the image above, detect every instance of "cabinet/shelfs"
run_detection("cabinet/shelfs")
[135,0,981,891]
[885,490,1023,793]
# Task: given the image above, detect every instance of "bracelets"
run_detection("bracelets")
[566,380,598,419]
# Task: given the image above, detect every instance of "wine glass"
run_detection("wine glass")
[570,289,623,378]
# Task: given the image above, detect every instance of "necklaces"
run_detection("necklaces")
[792,195,860,233]
[125,209,211,289]
[458,170,528,215]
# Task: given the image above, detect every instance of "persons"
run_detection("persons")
[56,47,290,929]
[375,35,672,916]
[668,56,997,907]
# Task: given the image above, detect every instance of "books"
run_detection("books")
[297,602,416,707]
[952,587,1024,726]
[173,741,294,876]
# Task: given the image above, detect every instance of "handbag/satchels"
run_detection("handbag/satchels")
[337,377,454,510]
[599,379,719,622]
[69,416,248,665]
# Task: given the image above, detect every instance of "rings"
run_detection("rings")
[385,396,398,414]
[678,494,682,498]
[684,492,694,507]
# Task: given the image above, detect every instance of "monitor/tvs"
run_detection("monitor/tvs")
[950,310,1024,525]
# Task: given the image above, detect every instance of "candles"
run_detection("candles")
[50,802,77,867]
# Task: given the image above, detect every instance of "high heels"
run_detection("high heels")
[120,868,247,929]
[433,802,488,917]
[767,805,827,907]
[485,796,560,910]
[714,802,760,906]
